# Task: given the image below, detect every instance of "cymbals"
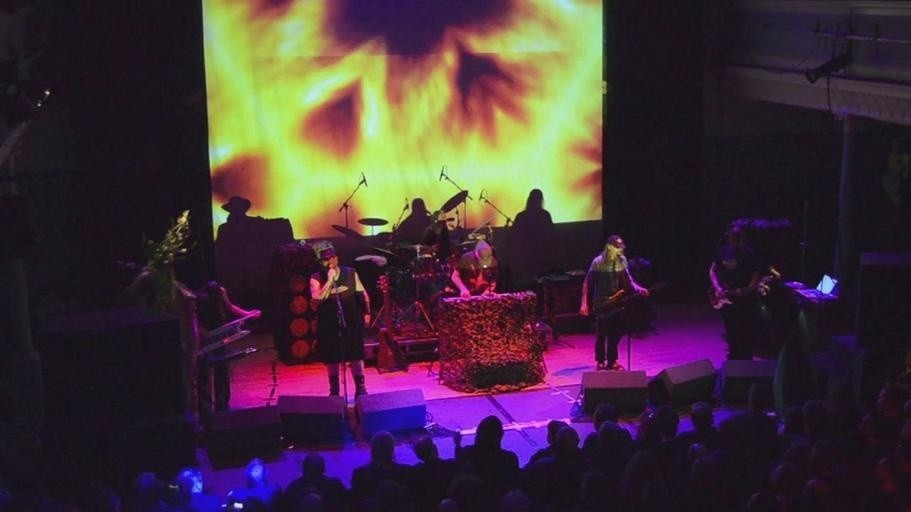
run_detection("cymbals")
[373,246,401,258]
[442,190,467,212]
[358,219,388,225]
[400,244,430,251]
[331,224,365,241]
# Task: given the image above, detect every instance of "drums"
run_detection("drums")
[427,287,460,332]
[411,253,448,281]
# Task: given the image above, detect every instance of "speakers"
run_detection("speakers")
[722,359,777,408]
[357,388,427,438]
[206,406,284,471]
[276,395,346,445]
[581,371,648,418]
[649,359,717,409]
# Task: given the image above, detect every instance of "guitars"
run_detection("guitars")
[376,274,403,369]
[591,281,666,322]
[708,274,775,311]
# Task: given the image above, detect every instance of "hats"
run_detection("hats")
[475,240,492,266]
[221,196,250,211]
[320,247,337,260]
[607,235,626,249]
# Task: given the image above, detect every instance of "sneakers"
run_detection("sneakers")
[606,361,624,371]
[596,361,604,372]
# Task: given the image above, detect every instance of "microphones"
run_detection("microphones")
[616,252,625,262]
[360,172,367,186]
[329,263,337,286]
[439,167,445,182]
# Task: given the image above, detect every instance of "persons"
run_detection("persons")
[214,336,911,511]
[576,233,653,371]
[704,217,774,361]
[110,188,560,449]
